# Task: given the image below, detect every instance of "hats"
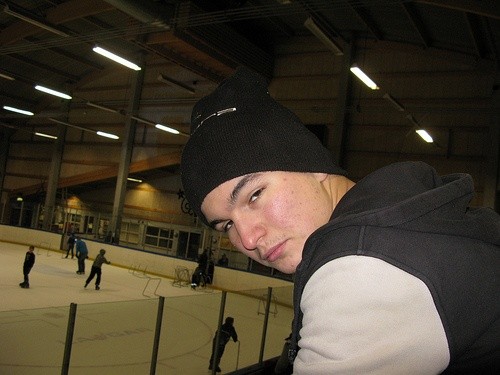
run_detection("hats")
[180,67,353,228]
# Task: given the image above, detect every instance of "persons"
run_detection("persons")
[105,231,114,243]
[190,248,228,288]
[65,233,76,259]
[75,236,88,274]
[180,67,500,375]
[209,317,237,373]
[84,249,111,290]
[19,245,35,288]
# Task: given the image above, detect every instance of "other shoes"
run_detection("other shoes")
[76,268,86,274]
[208,365,222,372]
[19,282,30,289]
[84,284,88,288]
[95,286,100,290]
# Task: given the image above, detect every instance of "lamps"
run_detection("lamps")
[85,100,129,119]
[96,130,120,141]
[32,81,73,102]
[382,92,406,112]
[92,42,143,71]
[349,62,380,91]
[303,15,345,56]
[154,123,180,135]
[155,72,196,95]
[407,114,442,149]
